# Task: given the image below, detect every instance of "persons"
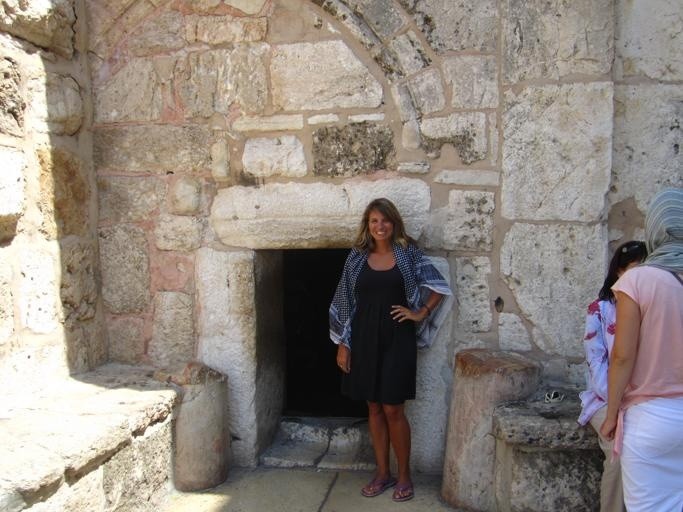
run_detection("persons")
[600,188,683,511]
[578,240,648,511]
[329,198,453,501]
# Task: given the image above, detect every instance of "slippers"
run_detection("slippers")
[360,478,398,497]
[392,479,414,502]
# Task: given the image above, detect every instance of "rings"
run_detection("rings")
[340,364,342,367]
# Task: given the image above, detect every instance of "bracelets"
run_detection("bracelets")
[421,304,431,316]
